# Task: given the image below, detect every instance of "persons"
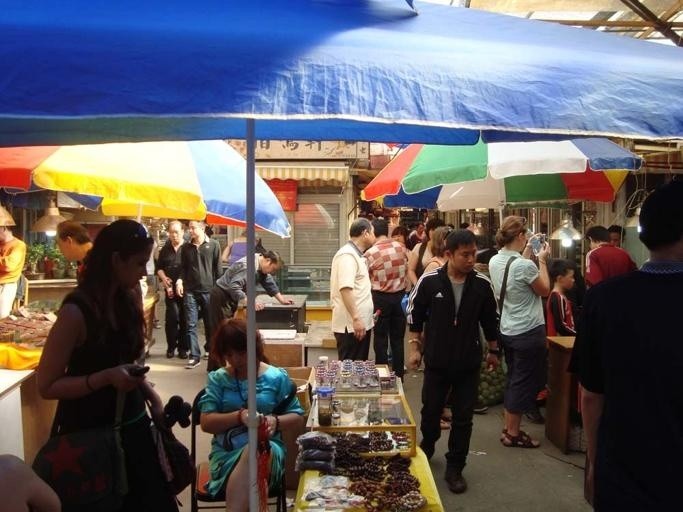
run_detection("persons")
[0,452,63,512]
[328,217,501,382]
[148,219,259,368]
[199,318,304,511]
[488,215,639,446]
[0,207,26,320]
[206,249,293,372]
[56,221,94,285]
[567,176,683,511]
[405,229,501,492]
[35,217,181,512]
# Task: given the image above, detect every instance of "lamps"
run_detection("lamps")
[545,208,581,249]
[28,192,67,237]
[472,211,488,236]
[622,160,646,233]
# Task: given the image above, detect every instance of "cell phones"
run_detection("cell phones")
[130,366,150,377]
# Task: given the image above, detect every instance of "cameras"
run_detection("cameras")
[540,234,547,243]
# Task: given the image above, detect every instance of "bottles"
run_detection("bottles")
[314,384,335,428]
[389,371,396,389]
[314,358,379,389]
[524,229,542,255]
[380,380,389,390]
[166,279,173,299]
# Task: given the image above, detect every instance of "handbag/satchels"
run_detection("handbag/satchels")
[31,433,112,505]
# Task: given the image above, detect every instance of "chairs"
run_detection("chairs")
[189,386,288,512]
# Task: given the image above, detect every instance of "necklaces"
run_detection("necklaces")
[233,369,249,402]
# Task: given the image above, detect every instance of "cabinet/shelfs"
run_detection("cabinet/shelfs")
[232,292,341,382]
[294,353,450,511]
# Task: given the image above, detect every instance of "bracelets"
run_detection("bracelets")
[85,374,94,391]
[237,407,245,425]
[272,413,280,435]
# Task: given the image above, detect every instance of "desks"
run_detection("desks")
[544,333,587,456]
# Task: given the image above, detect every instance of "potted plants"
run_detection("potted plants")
[22,241,78,280]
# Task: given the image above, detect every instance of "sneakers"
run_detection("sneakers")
[500,429,540,448]
[179,352,187,359]
[444,467,467,492]
[203,352,209,360]
[185,359,200,369]
[167,352,174,357]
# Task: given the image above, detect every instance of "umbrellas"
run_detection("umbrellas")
[0,1,681,512]
[356,136,644,226]
[0,139,294,238]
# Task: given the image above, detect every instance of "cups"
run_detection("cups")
[330,395,382,427]
